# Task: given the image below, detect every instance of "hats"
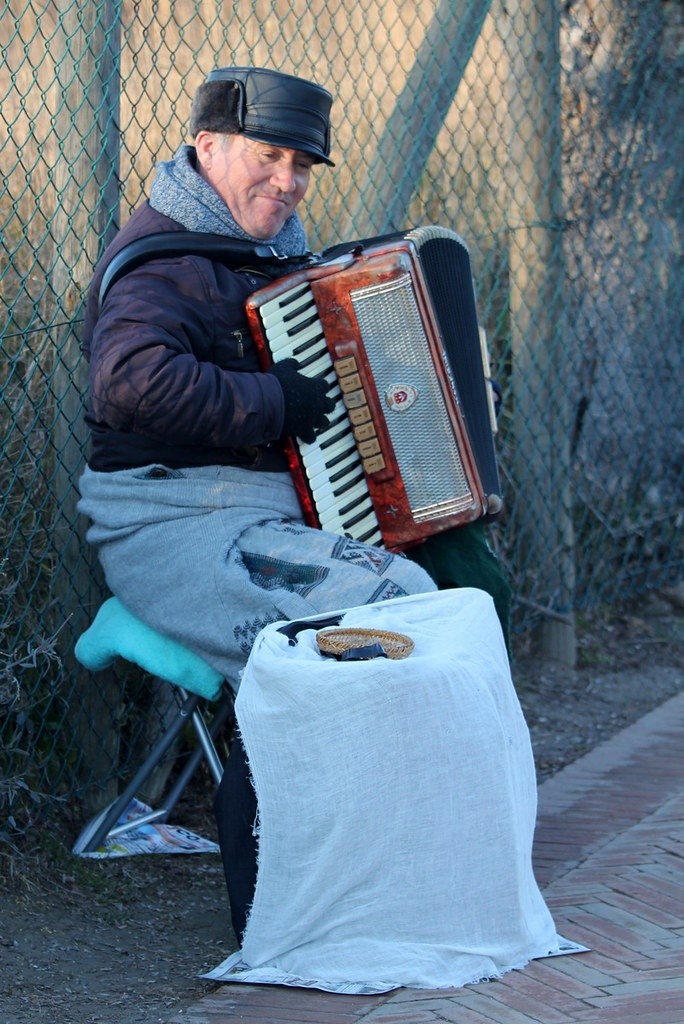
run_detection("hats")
[190,66,336,168]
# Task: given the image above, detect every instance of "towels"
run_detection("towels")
[72,595,226,703]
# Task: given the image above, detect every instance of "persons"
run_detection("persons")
[78,66,529,691]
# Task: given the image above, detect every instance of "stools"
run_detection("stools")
[75,596,224,857]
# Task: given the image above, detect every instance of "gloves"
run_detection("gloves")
[269,357,336,444]
